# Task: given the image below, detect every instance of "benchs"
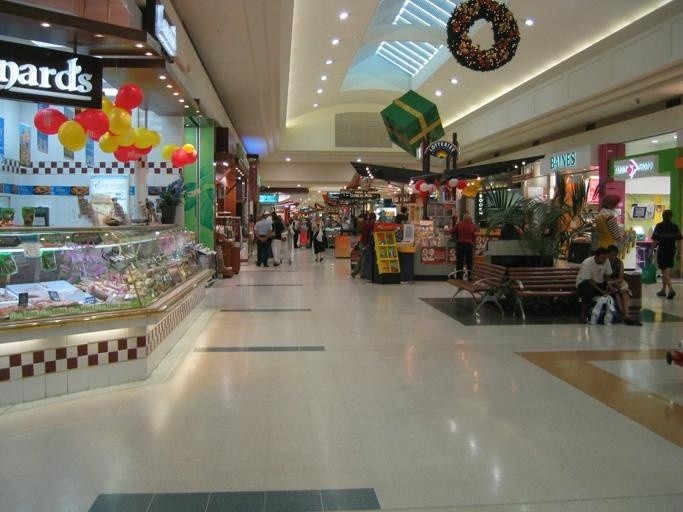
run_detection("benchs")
[506,265,625,323]
[447,262,507,318]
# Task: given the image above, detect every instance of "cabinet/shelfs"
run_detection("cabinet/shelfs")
[0,221,216,407]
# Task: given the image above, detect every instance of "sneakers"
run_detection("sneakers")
[657,290,667,296]
[667,290,676,299]
[256,261,280,267]
[314,258,322,262]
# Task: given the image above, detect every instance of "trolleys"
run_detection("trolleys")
[279,228,293,266]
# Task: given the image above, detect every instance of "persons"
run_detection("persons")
[248,209,337,268]
[647,210,683,299]
[449,214,476,281]
[103,198,163,224]
[344,207,409,279]
[575,247,618,324]
[607,244,633,320]
[588,194,625,256]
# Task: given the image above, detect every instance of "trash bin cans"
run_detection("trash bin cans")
[624,268,643,315]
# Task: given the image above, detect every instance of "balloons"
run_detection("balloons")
[415,177,465,198]
[161,143,197,168]
[33,83,161,162]
